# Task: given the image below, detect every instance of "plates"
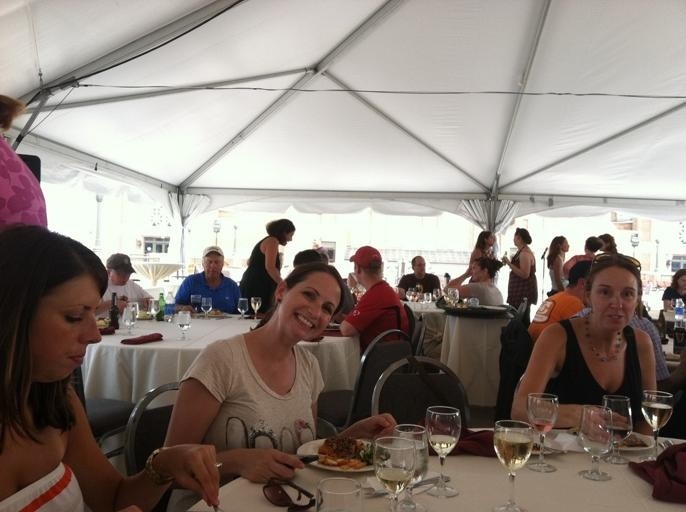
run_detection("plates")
[296,436,374,473]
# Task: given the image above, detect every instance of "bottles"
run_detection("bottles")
[502,251,507,265]
[673,298,685,329]
[107,292,120,330]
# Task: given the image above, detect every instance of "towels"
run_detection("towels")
[630,441,686,502]
[98,326,115,335]
[429,426,547,459]
[121,333,163,344]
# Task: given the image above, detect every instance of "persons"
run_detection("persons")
[2,224,221,512]
[93,253,154,316]
[165,219,685,512]
[1,96,48,227]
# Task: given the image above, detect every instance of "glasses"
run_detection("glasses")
[263,477,316,512]
[591,253,641,274]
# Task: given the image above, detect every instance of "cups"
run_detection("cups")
[317,477,362,511]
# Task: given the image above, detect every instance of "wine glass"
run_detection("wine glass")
[493,420,533,510]
[122,294,263,340]
[601,394,632,464]
[525,394,559,473]
[375,437,416,512]
[577,405,615,482]
[427,405,461,498]
[641,390,673,463]
[392,424,428,512]
[405,286,459,303]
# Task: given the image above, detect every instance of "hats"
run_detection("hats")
[203,246,224,257]
[106,253,136,276]
[349,246,382,269]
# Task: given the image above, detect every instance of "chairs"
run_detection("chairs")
[503,297,528,321]
[123,381,179,512]
[317,329,413,439]
[84,398,136,460]
[370,357,470,427]
